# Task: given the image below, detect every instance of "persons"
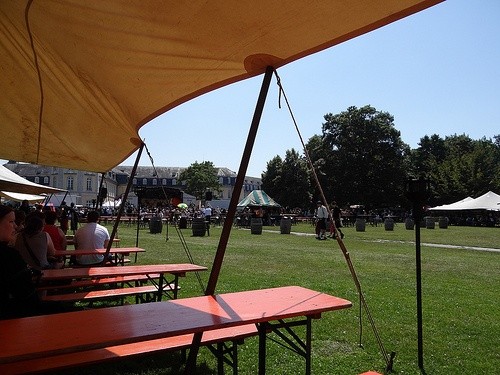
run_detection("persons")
[0,198,500,320]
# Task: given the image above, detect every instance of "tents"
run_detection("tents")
[0,162,74,207]
[237,190,281,208]
[425,191,500,226]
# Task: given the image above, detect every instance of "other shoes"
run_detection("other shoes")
[317,236,325,240]
[327,233,344,239]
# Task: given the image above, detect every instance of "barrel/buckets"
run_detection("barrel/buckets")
[426,218,435,229]
[149,216,162,234]
[179,216,187,229]
[280,218,291,234]
[250,217,262,234]
[405,217,414,230]
[438,216,448,229]
[356,218,365,232]
[192,218,206,237]
[384,218,394,231]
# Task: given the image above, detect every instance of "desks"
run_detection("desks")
[32,263,209,306]
[55,238,147,266]
[0,285,353,375]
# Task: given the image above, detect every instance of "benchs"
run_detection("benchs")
[103,257,131,264]
[0,323,259,375]
[32,273,182,303]
